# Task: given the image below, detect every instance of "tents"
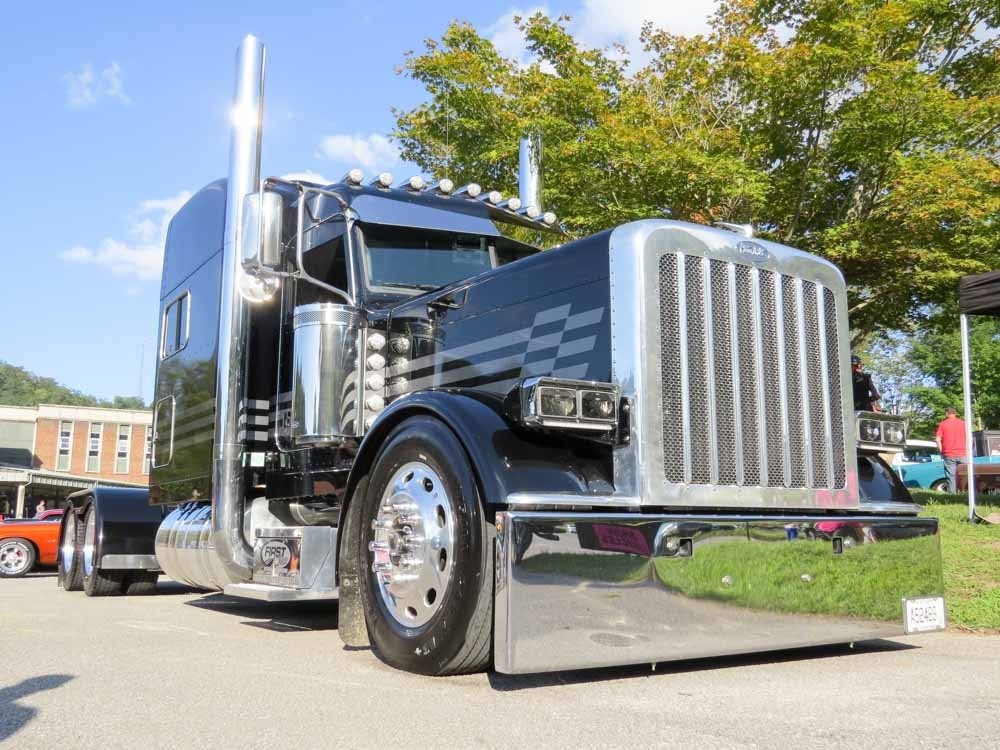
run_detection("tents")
[956,271,1000,522]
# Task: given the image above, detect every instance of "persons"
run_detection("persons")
[36,501,46,514]
[781,522,801,542]
[936,408,966,496]
[851,355,881,412]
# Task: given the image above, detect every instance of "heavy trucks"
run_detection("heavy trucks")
[55,35,946,676]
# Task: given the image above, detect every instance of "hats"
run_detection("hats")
[850,355,861,365]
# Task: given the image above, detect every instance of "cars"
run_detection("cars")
[1,509,64,578]
[879,431,1000,495]
